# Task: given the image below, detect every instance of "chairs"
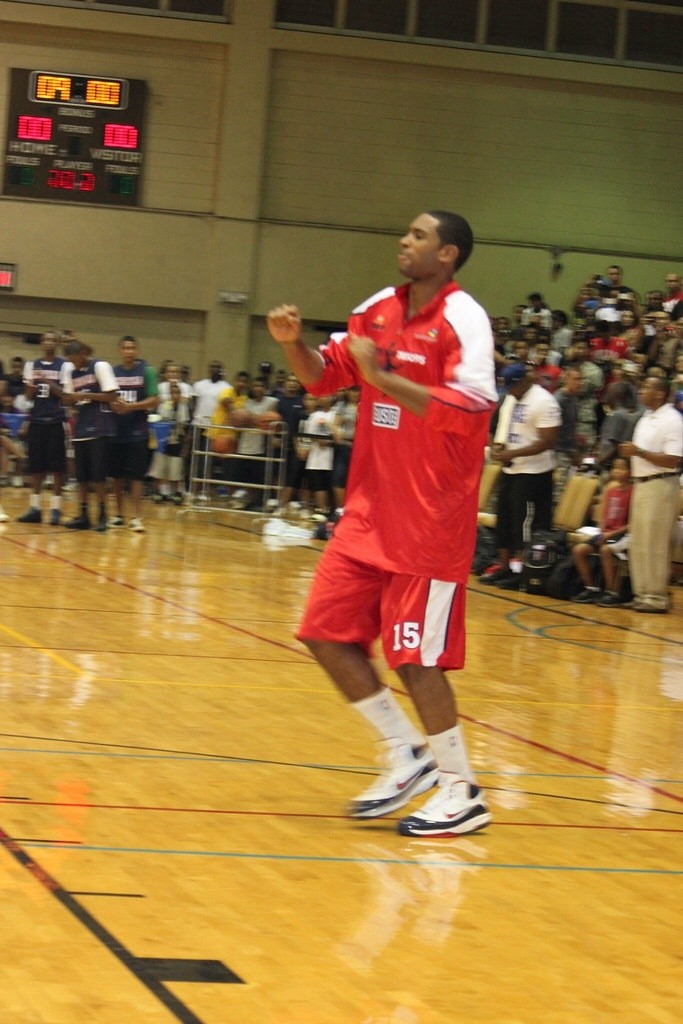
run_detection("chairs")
[477,464,501,526]
[551,475,620,537]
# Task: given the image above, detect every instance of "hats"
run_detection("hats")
[595,306,622,322]
[501,362,527,386]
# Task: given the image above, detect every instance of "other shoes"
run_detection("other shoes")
[7,474,344,521]
[17,507,42,522]
[96,518,107,532]
[573,586,601,602]
[634,598,669,612]
[126,518,146,531]
[65,517,90,529]
[597,590,624,606]
[51,511,60,525]
[112,516,123,525]
[0,509,9,521]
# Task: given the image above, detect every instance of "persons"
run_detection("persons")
[270,211,498,839]
[0,264,683,613]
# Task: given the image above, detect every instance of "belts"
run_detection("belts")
[627,471,682,484]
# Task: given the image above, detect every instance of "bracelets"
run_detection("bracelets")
[640,449,645,458]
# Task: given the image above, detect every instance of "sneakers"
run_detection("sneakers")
[346,742,442,818]
[396,780,492,838]
